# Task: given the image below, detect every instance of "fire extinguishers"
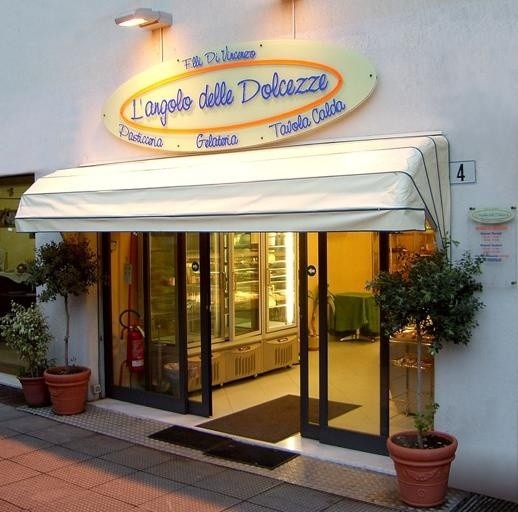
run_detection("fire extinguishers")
[119,310,145,373]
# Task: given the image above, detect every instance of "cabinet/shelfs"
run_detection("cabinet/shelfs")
[0,276,36,343]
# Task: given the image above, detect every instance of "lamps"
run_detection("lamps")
[115,7,170,31]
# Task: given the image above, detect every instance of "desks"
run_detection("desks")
[329,292,381,342]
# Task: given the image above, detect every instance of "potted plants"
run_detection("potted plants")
[308,284,336,351]
[0,301,58,409]
[27,239,100,414]
[365,237,483,503]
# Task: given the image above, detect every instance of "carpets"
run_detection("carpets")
[203,439,299,470]
[148,425,228,453]
[196,394,361,444]
